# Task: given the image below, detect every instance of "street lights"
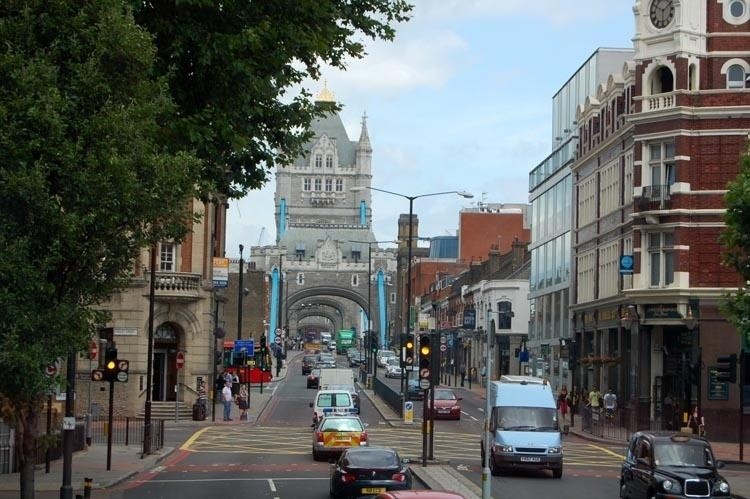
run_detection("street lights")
[238,241,244,374]
[284,275,306,337]
[348,185,476,391]
[330,237,404,351]
[279,252,303,329]
[482,307,514,499]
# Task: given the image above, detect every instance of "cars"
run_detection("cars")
[298,330,407,392]
[373,489,467,499]
[310,406,371,461]
[615,429,732,499]
[407,377,425,401]
[427,388,463,421]
[327,443,414,498]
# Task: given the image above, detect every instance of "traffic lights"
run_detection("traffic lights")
[407,334,414,357]
[105,347,118,382]
[418,333,431,369]
[260,335,267,356]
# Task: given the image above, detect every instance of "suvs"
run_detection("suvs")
[309,388,358,427]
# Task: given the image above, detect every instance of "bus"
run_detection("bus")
[224,340,274,383]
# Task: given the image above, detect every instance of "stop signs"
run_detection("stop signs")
[88,340,97,360]
[175,351,186,370]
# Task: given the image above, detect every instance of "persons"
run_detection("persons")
[199,380,209,420]
[222,380,234,421]
[217,370,240,402]
[687,404,705,436]
[556,382,675,442]
[237,383,249,420]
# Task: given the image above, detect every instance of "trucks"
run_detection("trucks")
[476,374,566,481]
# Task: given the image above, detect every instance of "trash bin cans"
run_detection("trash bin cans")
[192,403,205,421]
